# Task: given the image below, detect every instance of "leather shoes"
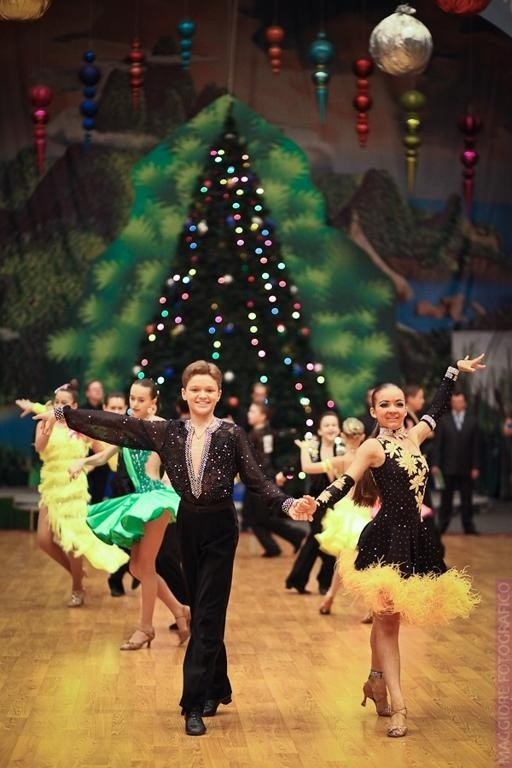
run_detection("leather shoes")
[108,574,125,598]
[184,706,206,735]
[67,569,88,607]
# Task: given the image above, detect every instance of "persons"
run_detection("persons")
[14,353,486,737]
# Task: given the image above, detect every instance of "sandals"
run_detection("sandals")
[170,603,191,647]
[387,704,408,739]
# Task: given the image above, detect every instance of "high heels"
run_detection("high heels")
[121,626,156,651]
[362,679,391,716]
[203,687,233,717]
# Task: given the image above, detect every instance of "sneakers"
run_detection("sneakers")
[360,609,373,624]
[293,528,308,554]
[316,575,329,596]
[286,577,311,595]
[319,592,336,614]
[131,576,141,590]
[260,548,283,558]
